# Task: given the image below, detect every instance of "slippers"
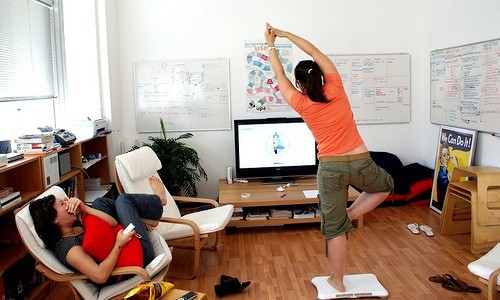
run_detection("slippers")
[420,225,435,236]
[406,222,420,234]
[442,279,481,293]
[428,273,454,283]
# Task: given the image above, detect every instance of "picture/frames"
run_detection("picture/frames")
[429,125,477,211]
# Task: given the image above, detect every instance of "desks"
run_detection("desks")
[218,177,365,235]
[110,288,208,300]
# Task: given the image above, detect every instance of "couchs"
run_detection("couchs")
[350,152,436,209]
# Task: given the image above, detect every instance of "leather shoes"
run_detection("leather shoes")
[214,280,251,297]
[220,274,239,283]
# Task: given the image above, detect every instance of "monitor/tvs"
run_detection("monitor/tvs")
[234,117,319,185]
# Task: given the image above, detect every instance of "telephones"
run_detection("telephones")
[52,127,78,146]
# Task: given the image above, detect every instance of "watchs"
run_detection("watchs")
[267,46,275,51]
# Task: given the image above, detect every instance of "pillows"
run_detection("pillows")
[468,241,500,284]
[80,213,144,268]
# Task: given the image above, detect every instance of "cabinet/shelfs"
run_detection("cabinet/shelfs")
[0,155,52,300]
[42,142,85,208]
[81,136,110,208]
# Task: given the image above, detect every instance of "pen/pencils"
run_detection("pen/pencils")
[279,194,287,198]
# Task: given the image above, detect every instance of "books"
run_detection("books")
[60,176,78,198]
[6,150,24,162]
[270,208,292,214]
[269,216,290,219]
[292,208,315,219]
[14,133,62,153]
[1,191,20,205]
[0,186,14,198]
[315,208,322,218]
[231,208,268,222]
[274,213,291,218]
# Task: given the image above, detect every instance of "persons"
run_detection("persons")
[271,132,285,155]
[437,142,458,211]
[29,175,167,288]
[264,21,396,293]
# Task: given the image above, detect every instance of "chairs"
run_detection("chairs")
[479,268,500,299]
[113,146,221,280]
[12,186,173,300]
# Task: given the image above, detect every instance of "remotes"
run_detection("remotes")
[176,292,197,300]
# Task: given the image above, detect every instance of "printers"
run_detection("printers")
[69,117,120,138]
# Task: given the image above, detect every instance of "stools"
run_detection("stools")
[441,193,491,255]
[440,181,500,245]
[450,165,500,226]
[441,188,500,250]
